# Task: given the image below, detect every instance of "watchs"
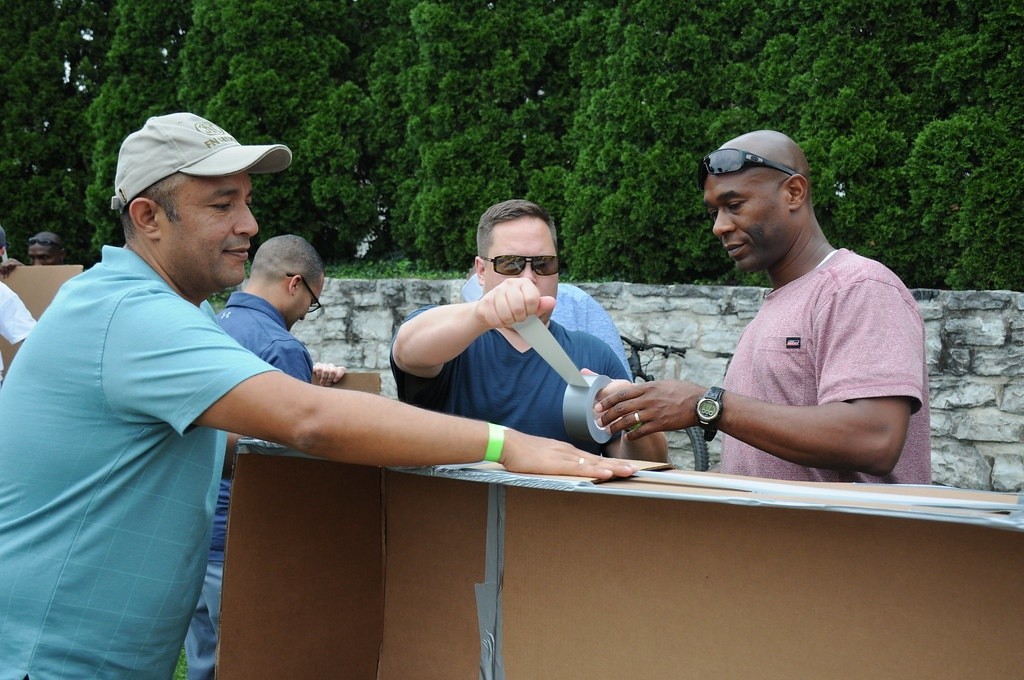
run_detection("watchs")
[695,385,726,442]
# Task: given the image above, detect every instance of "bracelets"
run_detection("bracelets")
[485,423,505,462]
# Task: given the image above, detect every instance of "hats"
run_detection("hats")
[111,113,292,213]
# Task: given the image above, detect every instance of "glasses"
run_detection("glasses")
[697,148,797,190]
[481,254,561,275]
[28,238,62,248]
[287,273,321,312]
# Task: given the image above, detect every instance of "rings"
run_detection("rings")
[634,412,640,424]
[578,457,584,469]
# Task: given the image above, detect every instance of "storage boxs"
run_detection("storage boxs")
[214,438,1024,680]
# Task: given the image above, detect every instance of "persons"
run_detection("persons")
[0,223,67,389]
[183,234,347,680]
[389,199,668,463]
[0,113,636,680]
[595,130,932,486]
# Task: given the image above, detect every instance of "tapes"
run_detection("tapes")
[561,374,614,448]
